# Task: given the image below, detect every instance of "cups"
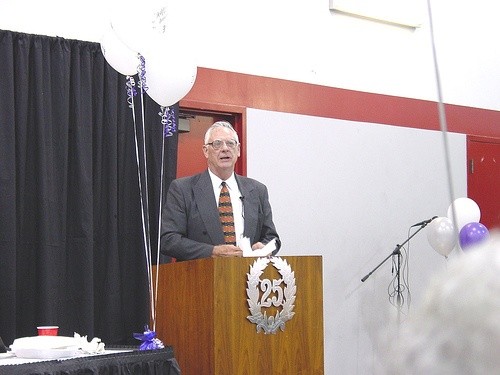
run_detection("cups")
[37,326,59,336]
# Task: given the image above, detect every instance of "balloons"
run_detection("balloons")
[426,197,492,259]
[101,0,199,110]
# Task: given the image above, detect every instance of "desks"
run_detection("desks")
[0,346,180,375]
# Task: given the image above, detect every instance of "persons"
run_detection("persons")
[385,238,500,375]
[157,117,283,263]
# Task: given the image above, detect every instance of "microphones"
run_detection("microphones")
[411,216,438,227]
[239,196,245,201]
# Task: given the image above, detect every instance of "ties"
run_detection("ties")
[217,182,237,248]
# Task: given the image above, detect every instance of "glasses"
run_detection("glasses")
[208,140,237,150]
